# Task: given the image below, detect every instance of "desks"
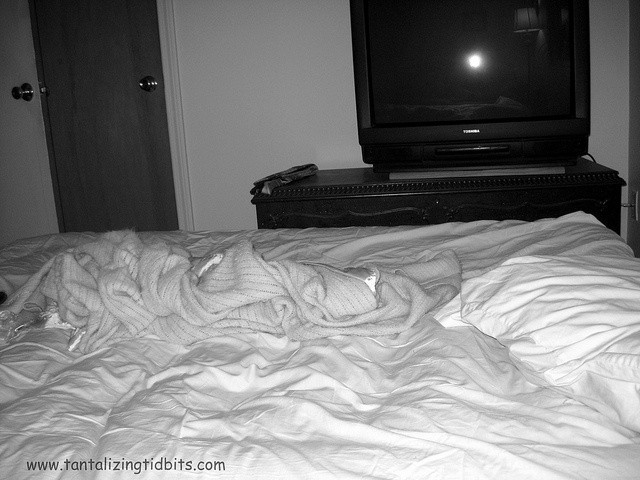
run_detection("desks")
[251,156,626,235]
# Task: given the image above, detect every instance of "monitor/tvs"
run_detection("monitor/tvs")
[350,0,591,174]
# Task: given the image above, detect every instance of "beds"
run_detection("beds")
[0,209,639,480]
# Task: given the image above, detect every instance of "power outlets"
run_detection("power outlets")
[632,192,638,221]
